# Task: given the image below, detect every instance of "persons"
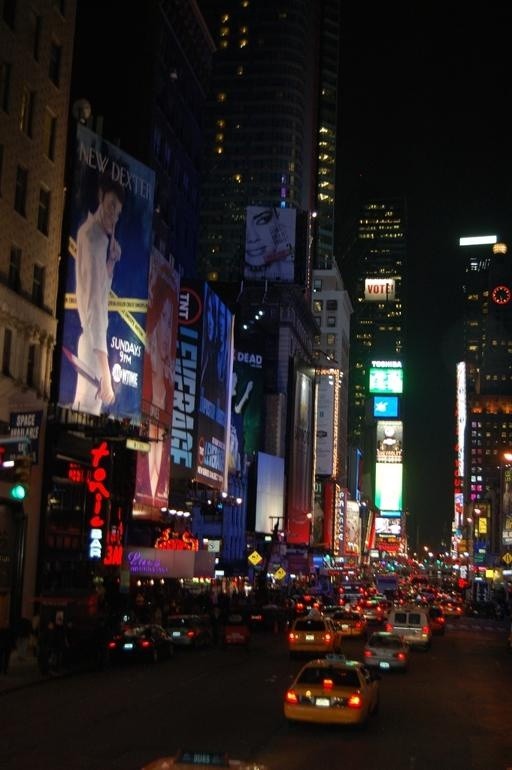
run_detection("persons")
[244,204,290,281]
[70,178,124,420]
[133,267,182,511]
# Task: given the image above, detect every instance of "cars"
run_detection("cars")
[166,614,214,649]
[140,751,268,769]
[215,597,279,632]
[363,631,410,670]
[282,653,380,726]
[427,608,447,632]
[295,581,467,622]
[331,612,366,636]
[107,622,176,663]
[287,607,342,653]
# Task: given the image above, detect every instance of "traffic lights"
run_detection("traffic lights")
[507,582,512,592]
[10,453,31,501]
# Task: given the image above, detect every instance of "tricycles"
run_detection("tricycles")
[224,608,253,644]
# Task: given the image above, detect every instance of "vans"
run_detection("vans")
[385,605,434,650]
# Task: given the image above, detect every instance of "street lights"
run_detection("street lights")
[467,515,476,552]
[497,451,512,552]
[473,501,482,551]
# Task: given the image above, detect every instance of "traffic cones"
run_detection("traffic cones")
[272,620,282,640]
